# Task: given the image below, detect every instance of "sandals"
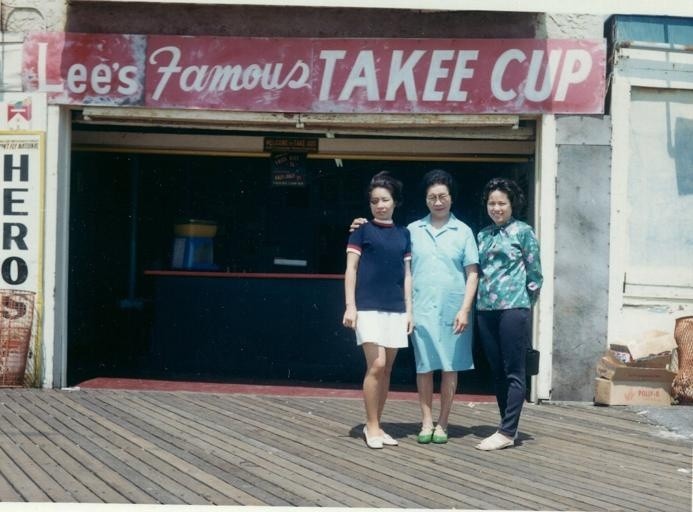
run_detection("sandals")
[417,424,449,444]
[363,424,398,449]
[475,427,519,450]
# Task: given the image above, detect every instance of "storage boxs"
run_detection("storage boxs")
[594,344,677,405]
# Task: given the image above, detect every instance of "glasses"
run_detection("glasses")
[427,193,451,202]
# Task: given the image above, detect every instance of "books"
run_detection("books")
[274,258,306,266]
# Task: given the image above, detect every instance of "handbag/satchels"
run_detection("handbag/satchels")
[526,350,540,376]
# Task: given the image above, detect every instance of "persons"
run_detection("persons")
[476,177,543,451]
[342,171,413,449]
[349,170,480,444]
